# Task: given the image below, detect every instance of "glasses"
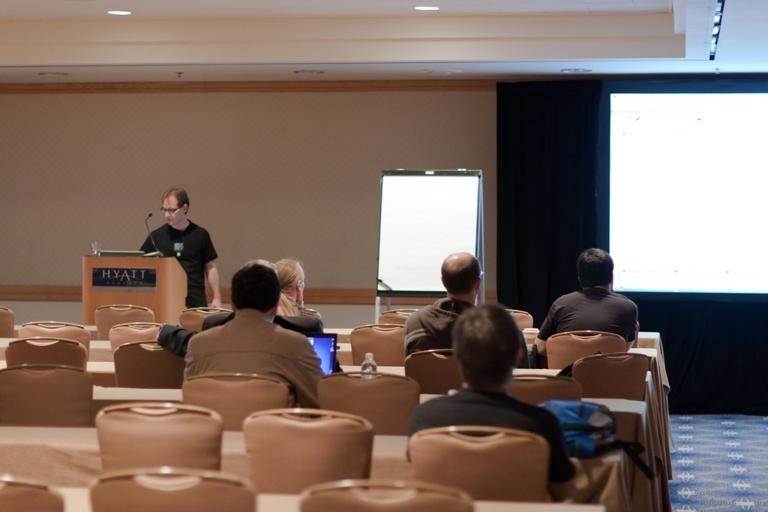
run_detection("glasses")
[160,207,179,214]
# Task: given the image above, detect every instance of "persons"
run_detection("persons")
[184,259,325,418]
[534,248,639,356]
[405,303,576,499]
[155,259,309,357]
[139,187,222,312]
[274,259,322,321]
[404,252,481,360]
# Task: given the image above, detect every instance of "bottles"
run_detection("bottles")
[357,352,380,378]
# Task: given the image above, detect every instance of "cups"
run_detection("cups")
[521,326,542,352]
[87,239,105,257]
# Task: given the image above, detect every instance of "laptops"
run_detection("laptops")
[303,334,338,377]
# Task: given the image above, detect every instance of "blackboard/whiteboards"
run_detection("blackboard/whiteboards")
[376,169,483,297]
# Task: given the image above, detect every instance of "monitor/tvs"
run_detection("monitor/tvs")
[99,249,145,256]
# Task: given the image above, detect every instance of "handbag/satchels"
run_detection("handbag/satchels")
[538,399,616,458]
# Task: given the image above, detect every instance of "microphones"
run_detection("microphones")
[144,211,166,259]
